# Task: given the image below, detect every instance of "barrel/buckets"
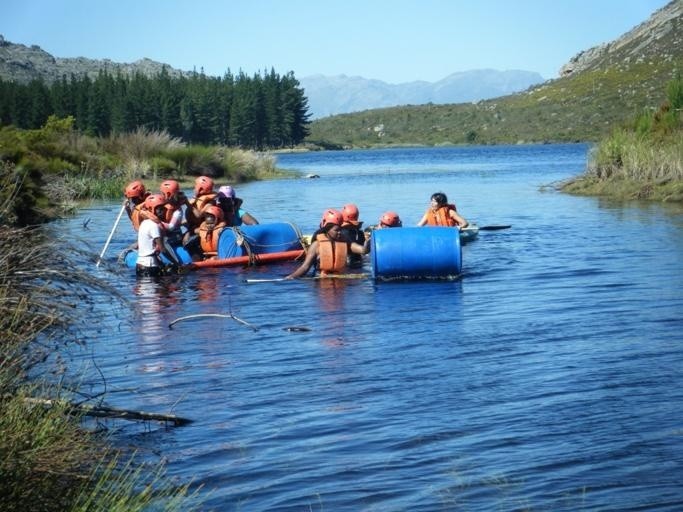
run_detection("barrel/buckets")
[123,247,193,267]
[370,226,462,278]
[217,222,303,258]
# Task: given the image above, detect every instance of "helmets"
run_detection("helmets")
[204,206,224,222]
[145,194,168,213]
[124,181,145,200]
[381,212,399,226]
[320,209,344,229]
[342,204,358,221]
[194,176,214,197]
[219,186,235,201]
[159,180,179,200]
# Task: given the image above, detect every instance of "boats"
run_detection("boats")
[300,222,482,280]
[121,220,306,269]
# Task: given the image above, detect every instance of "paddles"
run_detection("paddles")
[461,226,511,230]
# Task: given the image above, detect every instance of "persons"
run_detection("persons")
[137,194,164,276]
[311,209,351,265]
[336,204,367,268]
[417,192,468,230]
[215,185,258,227]
[160,179,191,245]
[191,206,226,259]
[124,182,150,232]
[188,176,215,226]
[287,209,369,279]
[363,211,402,238]
